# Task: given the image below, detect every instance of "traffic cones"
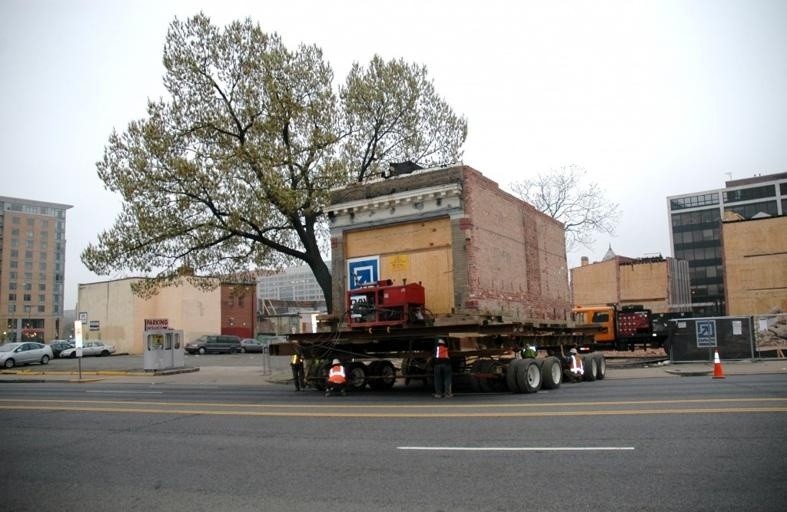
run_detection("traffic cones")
[711,347,726,379]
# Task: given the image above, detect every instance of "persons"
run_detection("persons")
[432,338,454,398]
[289,339,306,393]
[559,343,585,383]
[520,341,538,359]
[326,358,346,397]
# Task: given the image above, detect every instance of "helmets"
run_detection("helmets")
[331,358,342,365]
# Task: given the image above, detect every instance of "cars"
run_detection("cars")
[0,342,53,368]
[49,338,117,358]
[241,339,264,353]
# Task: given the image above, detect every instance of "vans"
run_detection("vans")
[185,336,241,353]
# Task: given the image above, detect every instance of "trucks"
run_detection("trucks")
[569,306,696,357]
